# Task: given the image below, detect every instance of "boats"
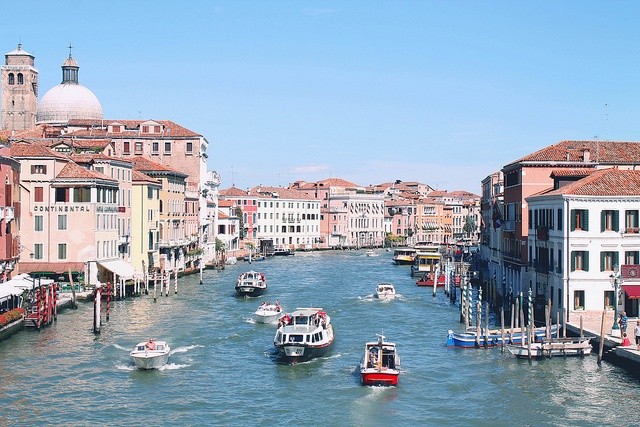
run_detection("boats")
[359,328,400,386]
[366,250,375,257]
[392,248,416,265]
[129,341,171,370]
[254,300,284,324]
[506,337,593,359]
[275,248,295,256]
[235,270,268,299]
[416,262,470,286]
[447,322,562,348]
[375,283,396,300]
[0,273,59,344]
[274,307,334,365]
[409,241,440,249]
[411,253,445,278]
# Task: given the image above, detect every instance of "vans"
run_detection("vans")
[29,271,58,283]
[62,271,80,282]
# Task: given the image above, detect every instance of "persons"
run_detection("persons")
[297,312,307,324]
[620,312,627,338]
[145,338,155,351]
[370,350,376,366]
[634,320,640,350]
[283,314,289,326]
[277,319,283,326]
[620,333,630,347]
[257,300,281,313]
[238,271,266,284]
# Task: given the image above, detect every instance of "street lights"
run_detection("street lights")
[610,263,624,330]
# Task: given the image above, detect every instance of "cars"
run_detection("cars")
[56,272,66,282]
